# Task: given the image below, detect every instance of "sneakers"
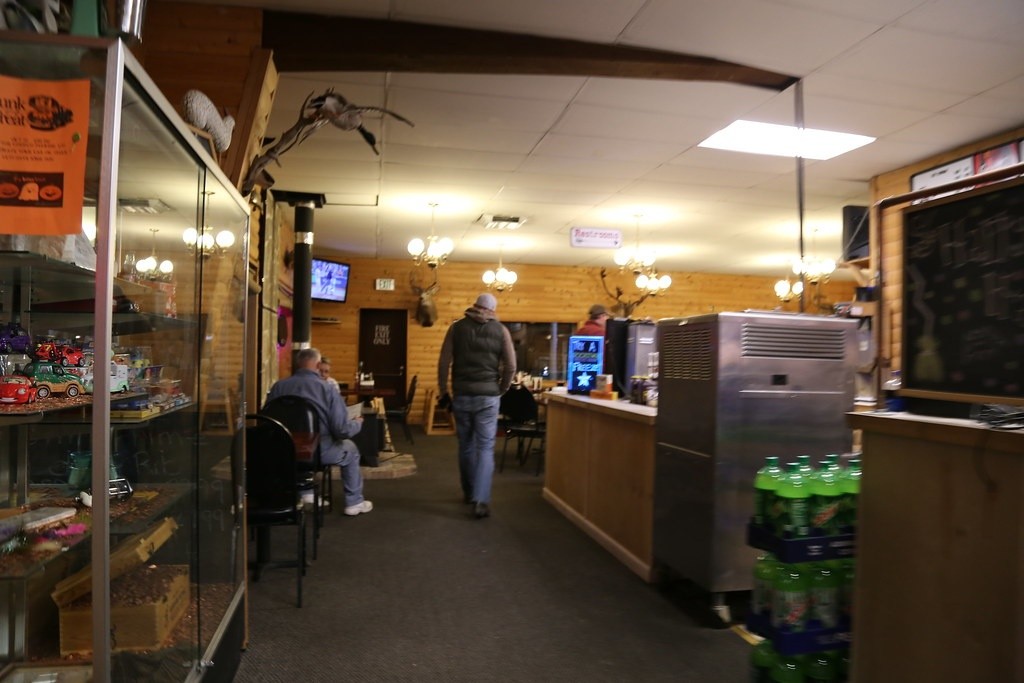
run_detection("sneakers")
[304,493,329,507]
[344,500,373,516]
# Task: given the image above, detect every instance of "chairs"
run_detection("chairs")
[500,381,547,476]
[245,395,333,608]
[383,376,418,445]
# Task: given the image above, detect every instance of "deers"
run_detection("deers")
[601,268,657,319]
[408,269,439,325]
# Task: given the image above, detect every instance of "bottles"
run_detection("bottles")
[843,460,864,533]
[796,455,815,483]
[750,549,853,637]
[630,375,659,406]
[825,454,841,476]
[751,640,854,683]
[775,464,811,539]
[754,456,787,527]
[884,371,902,412]
[811,461,844,538]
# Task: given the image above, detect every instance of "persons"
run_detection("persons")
[438,294,516,519]
[575,303,608,337]
[261,349,373,516]
[321,270,333,294]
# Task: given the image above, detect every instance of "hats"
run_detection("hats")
[589,305,606,316]
[476,293,497,312]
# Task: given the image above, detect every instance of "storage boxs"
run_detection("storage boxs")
[589,374,617,400]
[49,517,191,656]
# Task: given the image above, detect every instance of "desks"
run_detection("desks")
[254,430,321,580]
[528,385,547,405]
[339,387,398,398]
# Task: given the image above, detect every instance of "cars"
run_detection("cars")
[0,322,87,404]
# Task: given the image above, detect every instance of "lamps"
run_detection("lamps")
[774,252,836,302]
[482,248,518,293]
[613,242,674,296]
[181,189,235,259]
[135,227,174,277]
[408,200,452,269]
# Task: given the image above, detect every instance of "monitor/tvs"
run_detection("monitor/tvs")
[311,257,351,303]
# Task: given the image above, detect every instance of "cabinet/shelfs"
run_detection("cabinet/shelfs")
[0,28,252,683]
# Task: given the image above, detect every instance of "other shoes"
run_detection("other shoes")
[472,502,490,519]
[464,494,474,503]
